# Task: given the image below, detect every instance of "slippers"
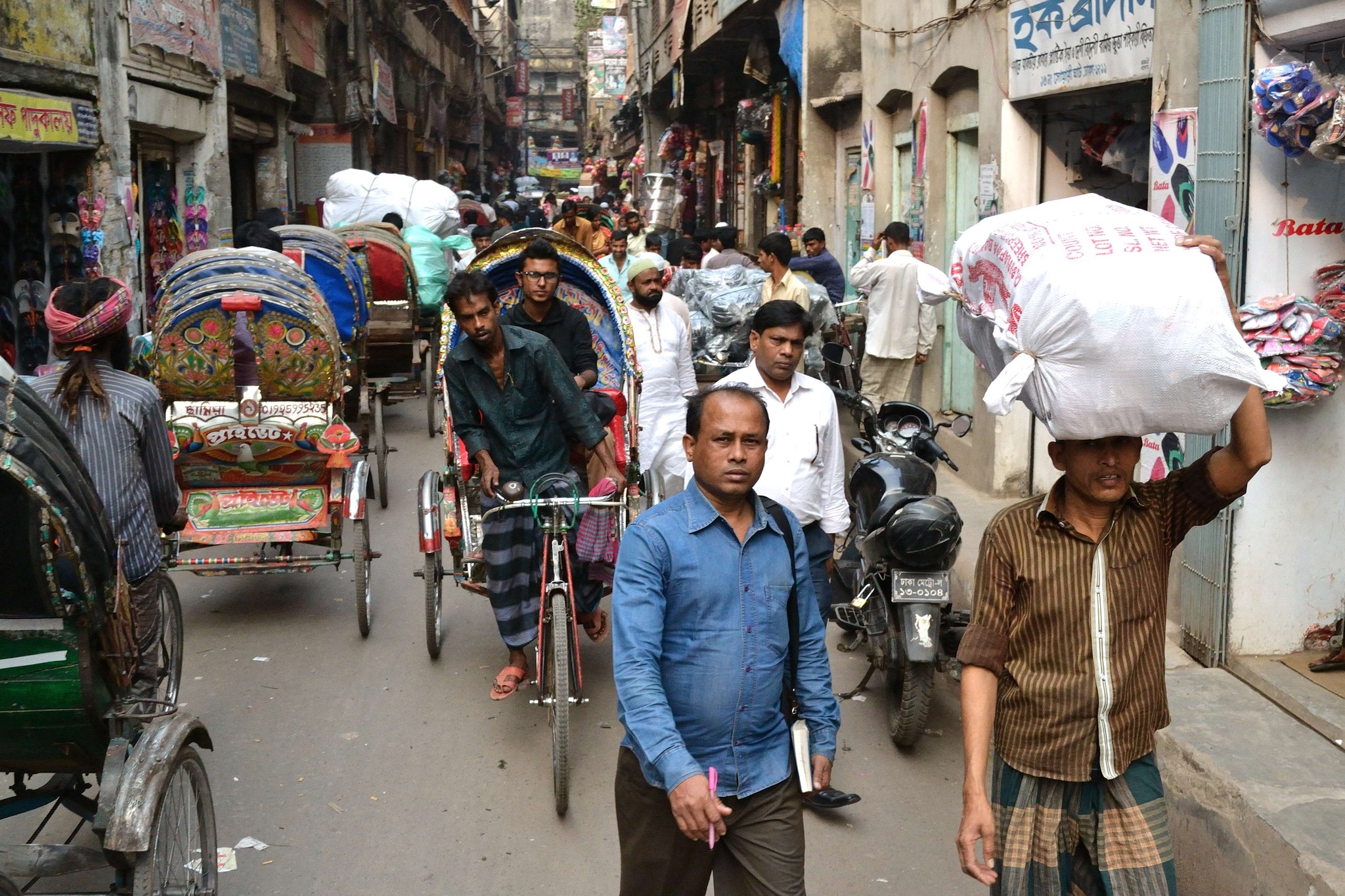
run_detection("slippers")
[490,663,528,700]
[581,606,609,644]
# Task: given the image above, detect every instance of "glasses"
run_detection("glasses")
[522,271,560,284]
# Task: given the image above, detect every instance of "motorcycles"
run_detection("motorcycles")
[818,342,973,752]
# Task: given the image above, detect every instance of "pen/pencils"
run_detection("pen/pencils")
[706,765,718,851]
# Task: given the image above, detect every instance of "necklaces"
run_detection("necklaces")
[636,305,664,354]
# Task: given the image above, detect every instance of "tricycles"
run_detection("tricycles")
[413,227,667,816]
[329,220,444,438]
[137,245,382,638]
[0,358,224,896]
[267,224,399,510]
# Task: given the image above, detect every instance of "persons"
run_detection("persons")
[609,385,846,896]
[755,231,809,375]
[219,170,761,301]
[25,275,190,723]
[619,251,698,507]
[781,227,845,312]
[443,272,627,701]
[852,221,940,440]
[710,297,852,627]
[952,231,1274,896]
[495,234,603,393]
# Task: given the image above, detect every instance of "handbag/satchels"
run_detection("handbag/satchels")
[780,667,800,728]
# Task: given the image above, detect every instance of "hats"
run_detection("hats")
[637,252,665,271]
[627,259,658,282]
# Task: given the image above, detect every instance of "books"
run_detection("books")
[790,718,814,794]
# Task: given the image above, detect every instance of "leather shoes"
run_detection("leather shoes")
[809,785,861,808]
